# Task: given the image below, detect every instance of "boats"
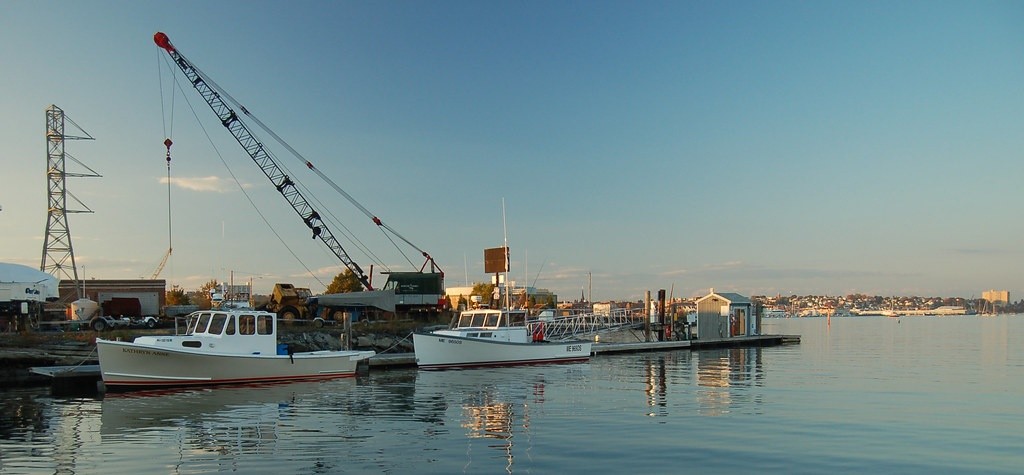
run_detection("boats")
[413,326,594,371]
[94,311,378,389]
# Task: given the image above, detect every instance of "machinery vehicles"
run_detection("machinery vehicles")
[149,29,450,329]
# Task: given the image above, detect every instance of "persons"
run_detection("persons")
[730,316,738,337]
